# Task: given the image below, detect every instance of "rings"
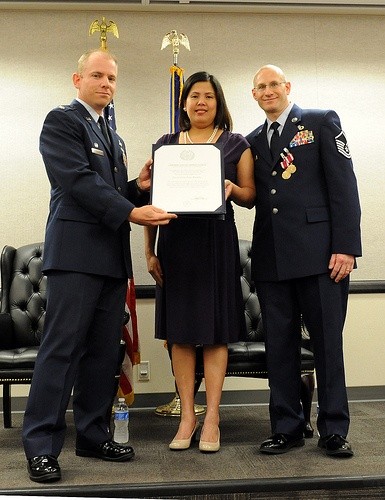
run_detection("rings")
[346,269,349,270]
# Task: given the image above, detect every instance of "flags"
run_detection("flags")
[102,100,141,406]
[164,66,186,349]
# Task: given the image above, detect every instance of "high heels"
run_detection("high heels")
[169,420,198,450]
[198,426,220,451]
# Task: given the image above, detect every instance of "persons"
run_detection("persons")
[22,50,177,481]
[144,71,256,451]
[245,64,361,457]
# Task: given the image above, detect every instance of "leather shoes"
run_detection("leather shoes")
[27,454,61,481]
[260,432,305,453]
[318,433,354,455]
[76,438,134,460]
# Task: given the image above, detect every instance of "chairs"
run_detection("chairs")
[0,243,130,427]
[195,239,316,438]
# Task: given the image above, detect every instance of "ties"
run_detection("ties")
[98,116,112,153]
[270,122,281,159]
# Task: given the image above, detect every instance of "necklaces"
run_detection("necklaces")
[185,128,218,144]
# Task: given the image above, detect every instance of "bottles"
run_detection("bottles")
[113,397,129,444]
